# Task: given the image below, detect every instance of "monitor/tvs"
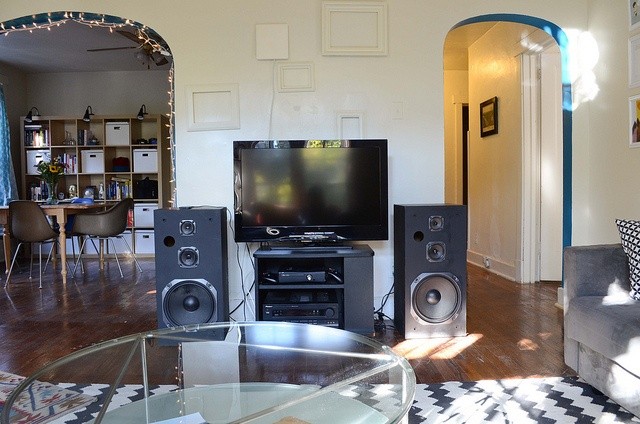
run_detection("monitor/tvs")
[233,138,390,250]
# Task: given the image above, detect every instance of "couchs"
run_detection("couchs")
[563,243,639,424]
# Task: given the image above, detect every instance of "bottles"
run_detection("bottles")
[98,184,104,201]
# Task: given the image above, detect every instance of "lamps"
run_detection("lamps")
[22,104,41,125]
[80,103,94,124]
[135,101,148,122]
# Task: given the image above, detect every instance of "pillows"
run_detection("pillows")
[612,217,640,299]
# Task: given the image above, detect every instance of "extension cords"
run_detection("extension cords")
[374,321,387,335]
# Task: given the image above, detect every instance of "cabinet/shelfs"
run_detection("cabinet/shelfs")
[20,114,172,257]
[255,243,375,336]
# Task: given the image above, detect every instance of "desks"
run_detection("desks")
[2,322,417,423]
[0,204,105,284]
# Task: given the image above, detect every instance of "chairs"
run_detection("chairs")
[73,196,143,278]
[3,199,75,292]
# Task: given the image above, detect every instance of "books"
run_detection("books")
[106,176,132,201]
[52,152,77,174]
[24,124,49,147]
[78,129,93,146]
[31,179,48,201]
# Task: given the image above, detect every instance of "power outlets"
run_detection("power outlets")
[481,255,491,273]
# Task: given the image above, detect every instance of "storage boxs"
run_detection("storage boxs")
[107,230,134,254]
[106,122,129,146]
[134,231,155,256]
[132,203,160,230]
[26,149,51,174]
[80,149,104,173]
[58,231,80,256]
[31,237,54,256]
[81,230,107,256]
[133,148,158,173]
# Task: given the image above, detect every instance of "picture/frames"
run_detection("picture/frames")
[183,79,244,133]
[626,91,640,149]
[317,0,390,62]
[626,28,640,93]
[336,108,370,145]
[275,59,317,96]
[478,96,499,140]
[625,0,640,29]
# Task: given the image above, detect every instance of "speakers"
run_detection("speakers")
[154,205,228,328]
[393,203,468,339]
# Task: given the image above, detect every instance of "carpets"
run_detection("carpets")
[1,368,98,424]
[44,374,640,423]
